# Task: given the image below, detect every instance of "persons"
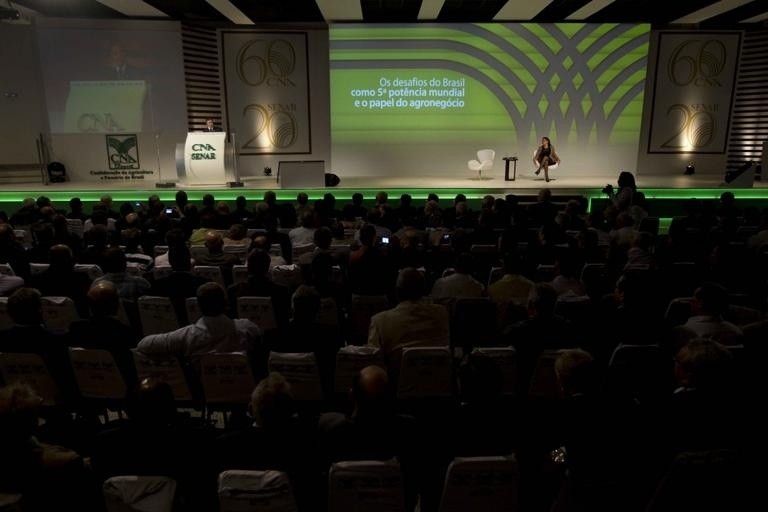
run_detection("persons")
[202,116,223,132]
[532,136,560,183]
[1,172,768,511]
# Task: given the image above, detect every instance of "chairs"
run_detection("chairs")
[0,209,768,512]
[468,149,495,180]
[533,149,558,181]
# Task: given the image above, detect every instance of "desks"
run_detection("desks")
[503,157,518,181]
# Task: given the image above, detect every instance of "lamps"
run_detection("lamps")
[46,160,66,183]
[263,166,272,175]
[684,164,695,174]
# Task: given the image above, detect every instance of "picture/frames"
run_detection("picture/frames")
[221,31,311,156]
[647,31,741,155]
